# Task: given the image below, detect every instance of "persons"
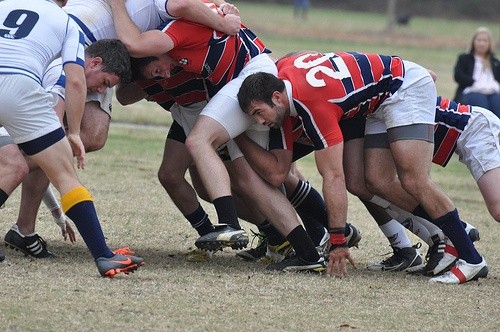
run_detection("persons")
[454,29,500,118]
[0,0,145,278]
[0,1,445,276]
[432,97,500,221]
[238,50,489,285]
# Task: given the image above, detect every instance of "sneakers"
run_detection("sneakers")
[4,229,52,259]
[429,257,488,285]
[195,223,249,252]
[435,220,480,276]
[265,240,296,265]
[95,247,145,278]
[423,239,448,275]
[188,246,220,261]
[367,242,424,273]
[265,254,325,274]
[315,222,361,261]
[236,228,268,262]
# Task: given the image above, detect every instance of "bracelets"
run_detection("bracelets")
[328,227,348,251]
